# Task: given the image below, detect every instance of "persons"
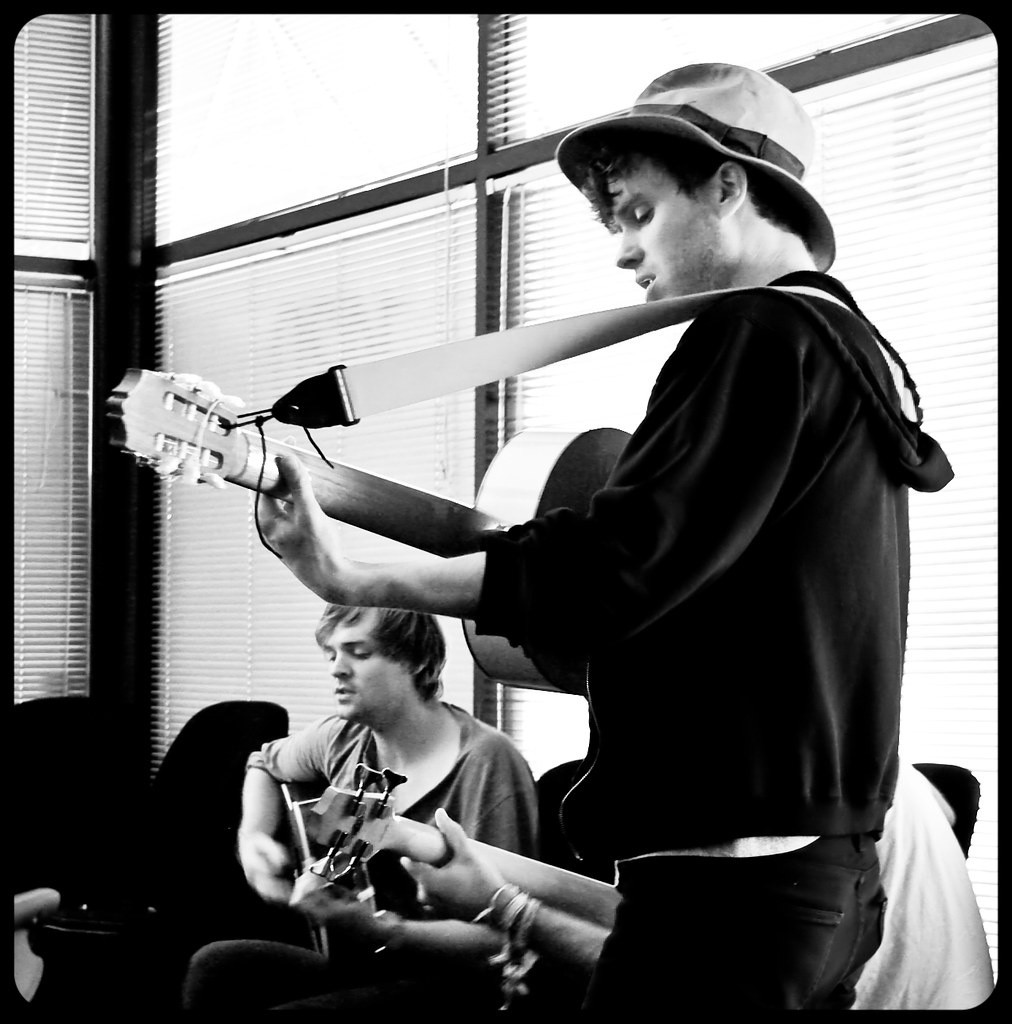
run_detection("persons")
[182,601,538,1024]
[254,63,953,1024]
[400,756,994,1012]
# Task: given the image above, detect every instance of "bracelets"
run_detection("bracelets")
[484,878,541,945]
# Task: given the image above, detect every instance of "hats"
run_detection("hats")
[555,62,834,274]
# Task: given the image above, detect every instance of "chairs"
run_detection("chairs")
[12,696,110,885]
[138,700,290,920]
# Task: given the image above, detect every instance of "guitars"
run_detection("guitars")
[308,760,618,929]
[278,780,375,957]
[110,365,632,696]
